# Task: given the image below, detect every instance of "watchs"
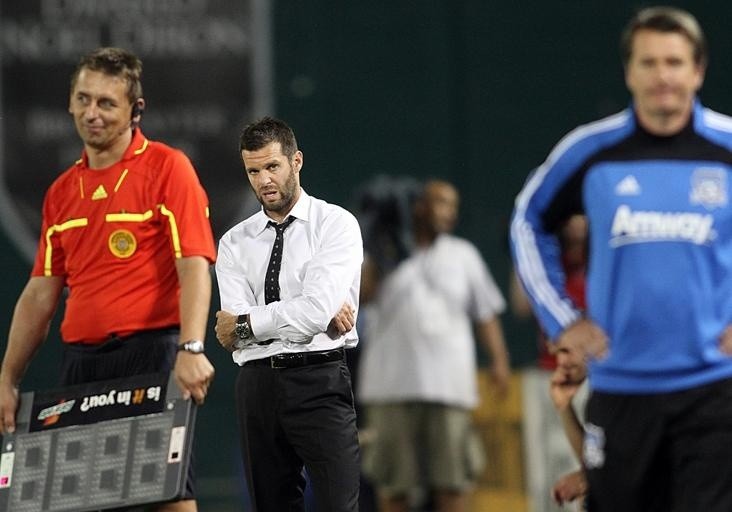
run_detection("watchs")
[177,339,206,355]
[235,314,251,340]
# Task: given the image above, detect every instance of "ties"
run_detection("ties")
[265,215,296,305]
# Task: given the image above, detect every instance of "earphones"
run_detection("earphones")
[131,104,143,119]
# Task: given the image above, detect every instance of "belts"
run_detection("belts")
[244,347,344,370]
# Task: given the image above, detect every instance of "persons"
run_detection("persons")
[494,207,595,510]
[499,5,732,510]
[208,111,368,509]
[1,46,219,512]
[338,177,514,512]
[546,317,590,512]
